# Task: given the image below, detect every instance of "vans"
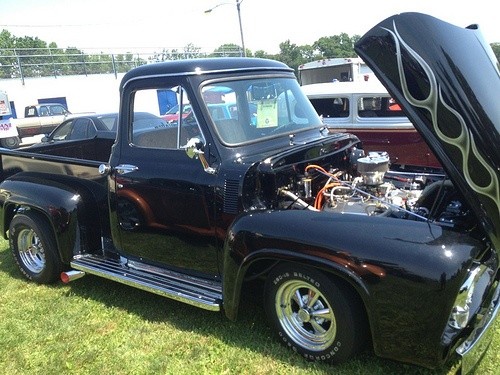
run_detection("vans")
[276,81,446,171]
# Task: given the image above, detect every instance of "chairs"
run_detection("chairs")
[337,111,349,118]
[133,127,190,155]
[213,118,249,144]
[359,110,377,117]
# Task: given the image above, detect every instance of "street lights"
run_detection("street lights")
[204,2,247,57]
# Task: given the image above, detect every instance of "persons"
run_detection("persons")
[332,78,343,112]
[363,74,373,110]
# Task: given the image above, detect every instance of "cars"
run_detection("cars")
[159,102,224,123]
[32,112,173,148]
[178,101,258,126]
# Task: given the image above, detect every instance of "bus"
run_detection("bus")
[297,56,394,110]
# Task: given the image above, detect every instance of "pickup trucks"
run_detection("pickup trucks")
[0,103,100,150]
[0,10,500,375]
